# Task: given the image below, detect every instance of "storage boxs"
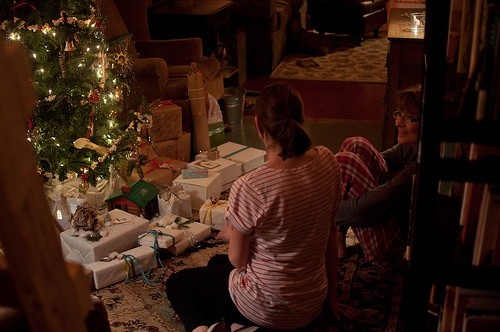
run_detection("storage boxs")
[49,98,267,290]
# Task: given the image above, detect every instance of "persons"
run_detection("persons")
[335,84,421,269]
[165,81,356,332]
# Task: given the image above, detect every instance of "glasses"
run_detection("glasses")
[392,111,419,123]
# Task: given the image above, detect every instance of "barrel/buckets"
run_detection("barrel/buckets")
[220,87,245,126]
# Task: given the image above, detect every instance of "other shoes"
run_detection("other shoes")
[206,322,227,332]
[240,326,260,332]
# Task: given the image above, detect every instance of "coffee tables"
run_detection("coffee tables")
[147,0,248,85]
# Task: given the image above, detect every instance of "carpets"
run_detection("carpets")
[269,29,390,84]
[92,224,404,332]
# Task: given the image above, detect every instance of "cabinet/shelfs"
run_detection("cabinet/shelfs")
[397,0,500,332]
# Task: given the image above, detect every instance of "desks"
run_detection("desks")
[381,9,425,151]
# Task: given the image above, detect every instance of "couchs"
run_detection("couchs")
[95,0,231,134]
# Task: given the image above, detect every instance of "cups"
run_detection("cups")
[410,13,425,33]
